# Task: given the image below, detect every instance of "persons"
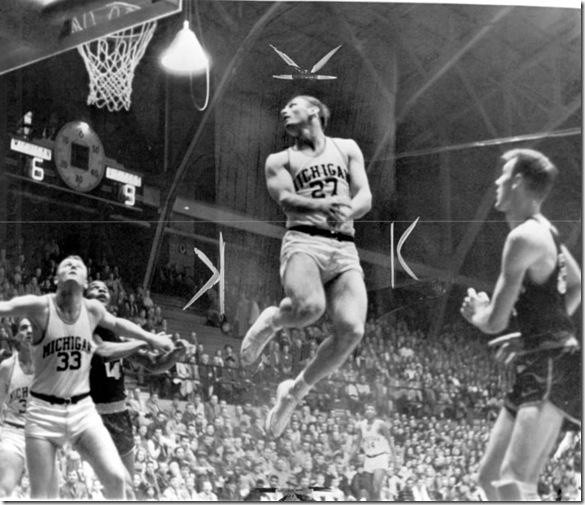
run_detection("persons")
[458,145,582,502]
[0,224,583,502]
[234,92,375,437]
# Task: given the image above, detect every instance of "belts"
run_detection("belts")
[30,390,91,406]
[287,225,353,241]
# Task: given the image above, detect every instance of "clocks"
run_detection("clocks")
[53,121,107,192]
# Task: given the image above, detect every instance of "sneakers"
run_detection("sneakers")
[266,379,298,441]
[241,307,279,366]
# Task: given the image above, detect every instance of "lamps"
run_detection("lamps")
[159,0,212,111]
